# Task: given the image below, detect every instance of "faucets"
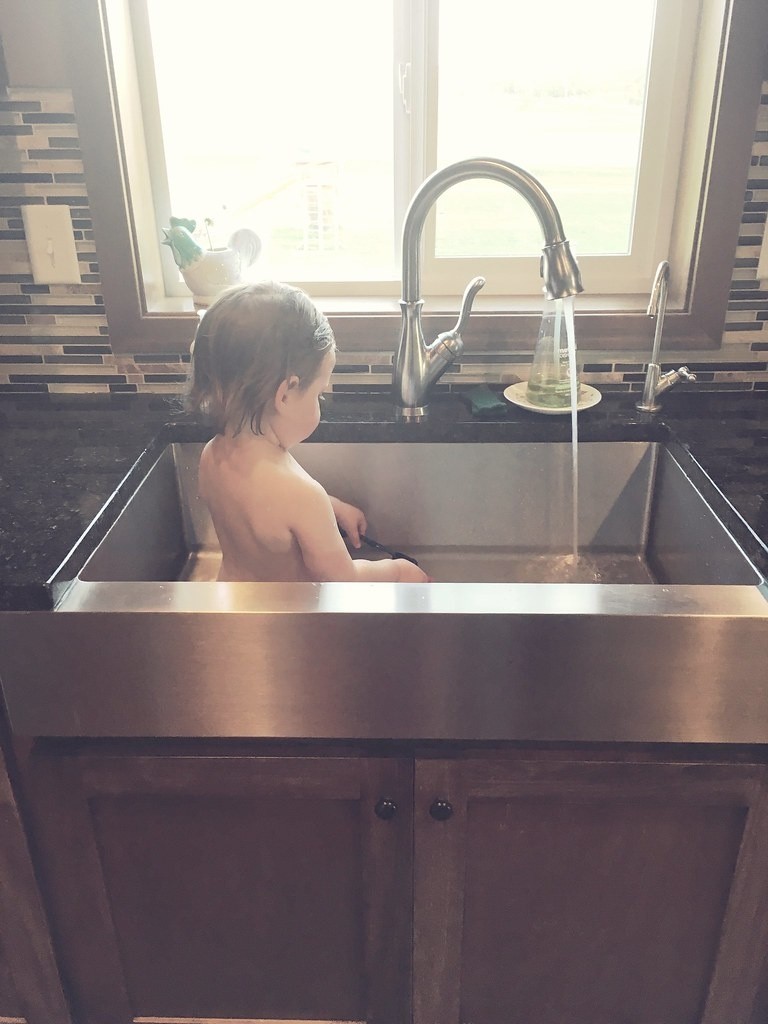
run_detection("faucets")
[392,157,583,418]
[632,260,697,414]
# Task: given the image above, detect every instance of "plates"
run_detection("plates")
[502,381,603,415]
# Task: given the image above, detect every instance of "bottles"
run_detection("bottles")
[525,286,581,409]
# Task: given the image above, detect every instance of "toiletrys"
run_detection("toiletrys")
[529,253,584,408]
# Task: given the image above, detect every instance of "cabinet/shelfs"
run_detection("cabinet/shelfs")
[14,732,768,1023]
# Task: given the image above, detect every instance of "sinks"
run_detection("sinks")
[77,431,762,585]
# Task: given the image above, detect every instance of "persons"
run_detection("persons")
[160,281,428,584]
